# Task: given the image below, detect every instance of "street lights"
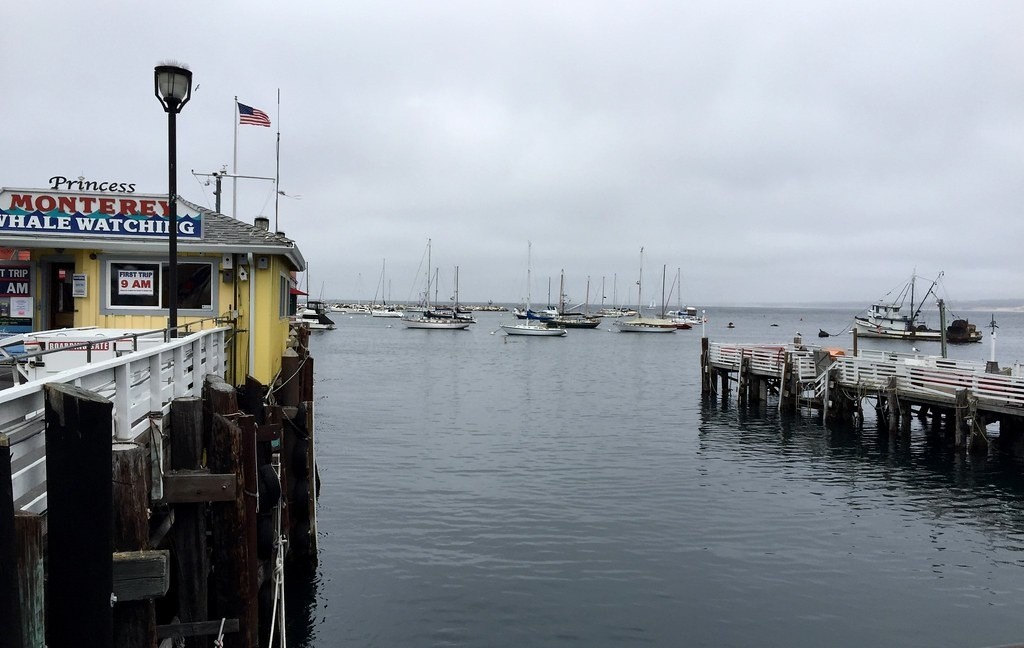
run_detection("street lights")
[152,59,194,337]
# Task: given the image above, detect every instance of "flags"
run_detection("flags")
[238,102,271,127]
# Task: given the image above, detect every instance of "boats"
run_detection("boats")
[852,268,986,343]
[509,267,639,329]
[296,256,478,324]
[293,310,336,330]
[655,261,707,331]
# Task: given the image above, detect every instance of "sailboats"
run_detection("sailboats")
[499,241,568,336]
[611,245,678,332]
[396,238,470,328]
